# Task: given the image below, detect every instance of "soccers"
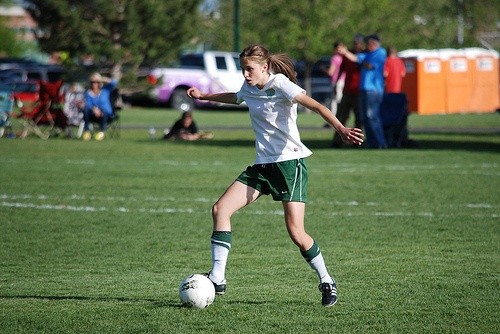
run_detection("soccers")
[179,274,216,310]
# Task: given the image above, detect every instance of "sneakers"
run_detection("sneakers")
[201,272,226,294]
[318,278,337,308]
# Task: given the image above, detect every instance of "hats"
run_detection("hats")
[364,35,380,42]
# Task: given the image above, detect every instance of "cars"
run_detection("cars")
[288,59,332,104]
[0,62,68,116]
[315,56,332,72]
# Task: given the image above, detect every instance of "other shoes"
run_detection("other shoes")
[94,132,105,141]
[82,131,91,140]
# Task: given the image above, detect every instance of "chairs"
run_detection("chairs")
[18,78,122,138]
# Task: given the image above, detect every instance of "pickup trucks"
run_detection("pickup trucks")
[120,50,248,112]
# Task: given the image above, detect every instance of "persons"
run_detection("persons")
[186,45,365,307]
[327,34,407,150]
[163,112,201,140]
[62,71,123,142]
